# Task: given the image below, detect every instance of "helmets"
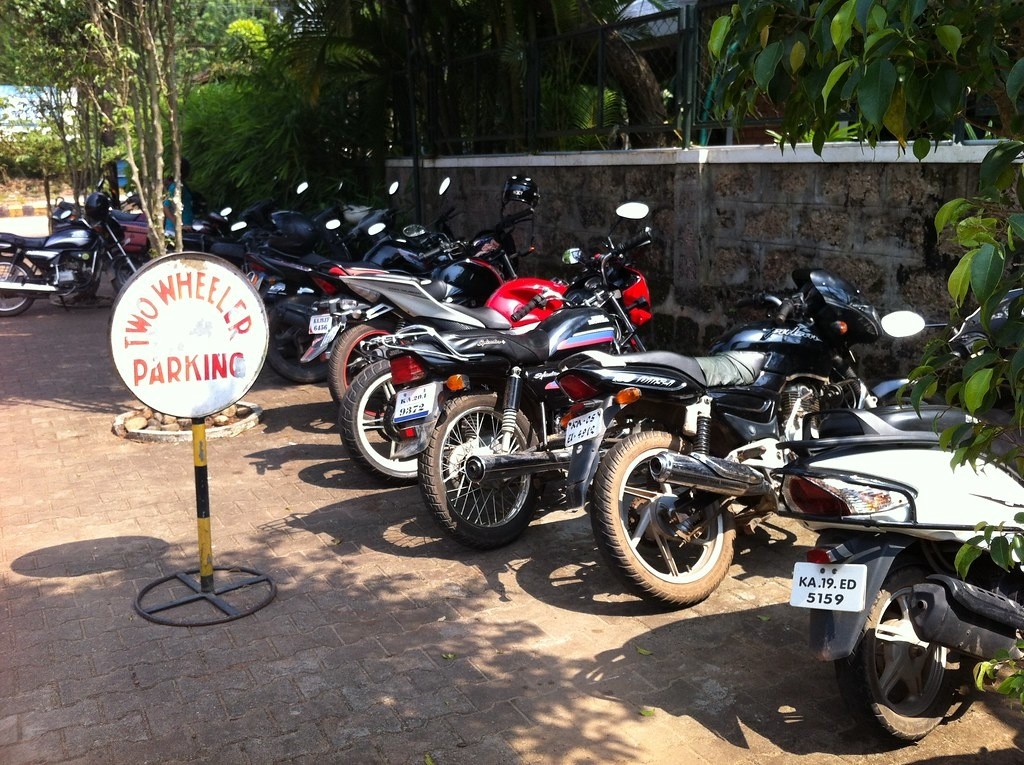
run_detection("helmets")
[501,174,539,208]
[85,192,110,219]
[268,210,320,257]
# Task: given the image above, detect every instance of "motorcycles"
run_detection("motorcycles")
[110,170,569,488]
[768,286,1024,744]
[0,178,145,317]
[340,200,655,550]
[554,267,946,610]
